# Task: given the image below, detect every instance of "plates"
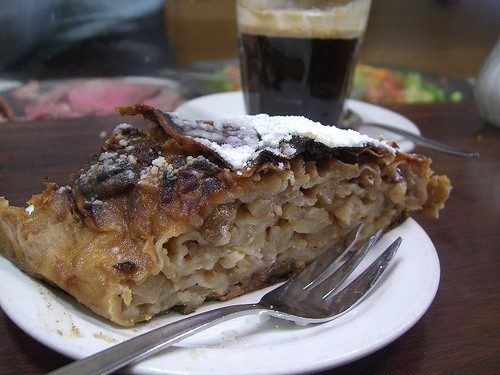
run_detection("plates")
[174,91,421,153]
[0,205,441,375]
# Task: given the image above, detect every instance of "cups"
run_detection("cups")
[236,0,372,127]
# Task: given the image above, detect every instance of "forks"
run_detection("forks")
[46,223,402,375]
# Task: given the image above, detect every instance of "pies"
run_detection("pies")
[0,102,453,326]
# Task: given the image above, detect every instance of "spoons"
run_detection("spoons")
[338,108,479,158]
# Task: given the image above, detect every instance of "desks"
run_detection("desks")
[0,101,500,375]
